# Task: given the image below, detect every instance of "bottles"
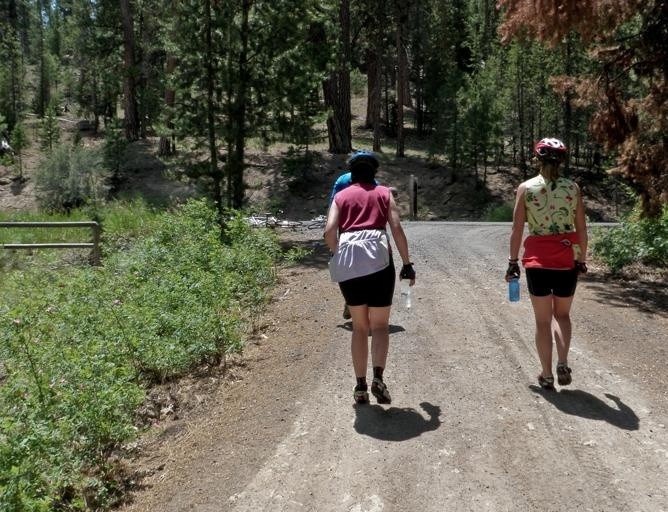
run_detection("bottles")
[507,274,522,303]
[397,274,413,310]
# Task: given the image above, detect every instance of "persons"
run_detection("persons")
[505,136,588,391]
[324,150,416,405]
[327,171,380,319]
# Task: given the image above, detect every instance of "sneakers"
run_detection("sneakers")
[352,384,369,404]
[343,304,351,319]
[537,374,555,389]
[556,362,572,386]
[371,377,392,404]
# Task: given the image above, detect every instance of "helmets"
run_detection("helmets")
[534,137,567,163]
[345,148,380,170]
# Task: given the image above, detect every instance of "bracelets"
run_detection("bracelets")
[509,257,519,263]
